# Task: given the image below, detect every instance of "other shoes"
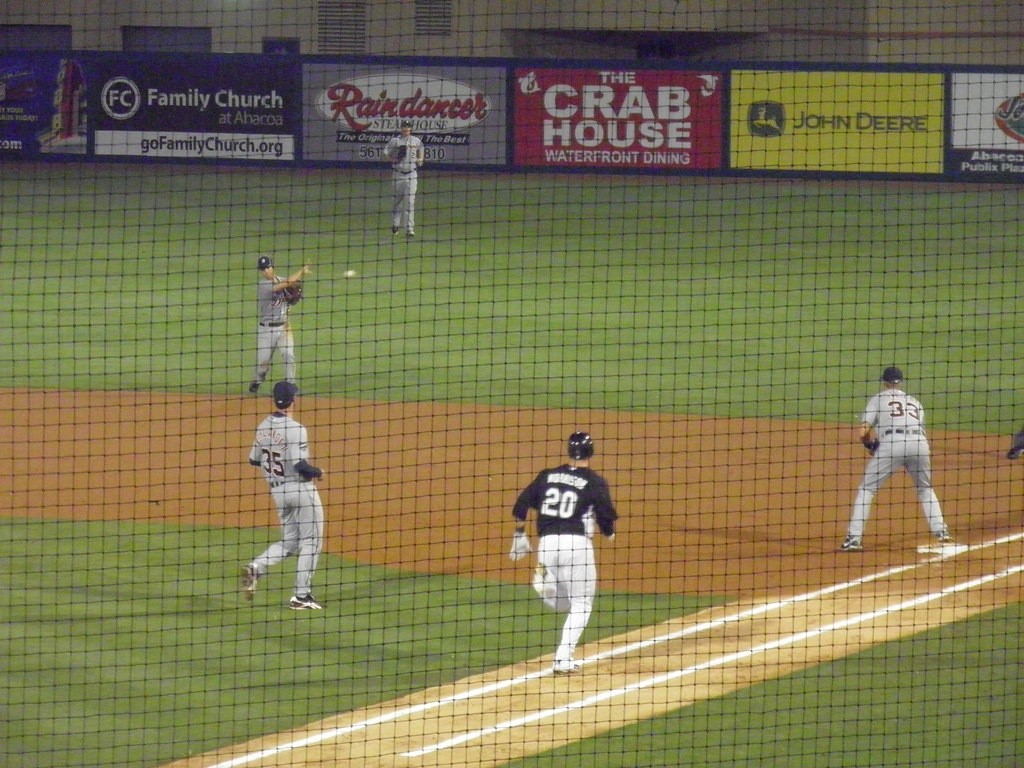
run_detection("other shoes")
[392,227,399,235]
[935,530,954,543]
[406,230,415,235]
[249,383,261,392]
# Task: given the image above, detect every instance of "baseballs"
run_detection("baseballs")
[345,270,354,278]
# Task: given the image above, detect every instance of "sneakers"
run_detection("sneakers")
[241,565,257,601]
[841,536,862,551]
[289,596,323,610]
[551,659,579,675]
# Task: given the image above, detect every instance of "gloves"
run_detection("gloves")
[509,532,532,561]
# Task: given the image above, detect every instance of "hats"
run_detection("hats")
[274,382,299,405]
[881,367,903,384]
[401,120,413,127]
[258,257,273,267]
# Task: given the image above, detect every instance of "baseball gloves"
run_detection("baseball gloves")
[283,284,302,305]
[864,438,879,454]
[397,145,406,161]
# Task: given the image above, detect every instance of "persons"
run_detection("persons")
[247,255,312,405]
[1007,422,1024,459]
[383,120,425,238]
[241,381,326,610]
[510,432,620,675]
[839,366,955,551]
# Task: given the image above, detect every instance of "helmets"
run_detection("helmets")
[568,432,594,460]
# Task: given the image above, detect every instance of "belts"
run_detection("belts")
[881,429,924,437]
[270,482,286,488]
[260,323,284,327]
